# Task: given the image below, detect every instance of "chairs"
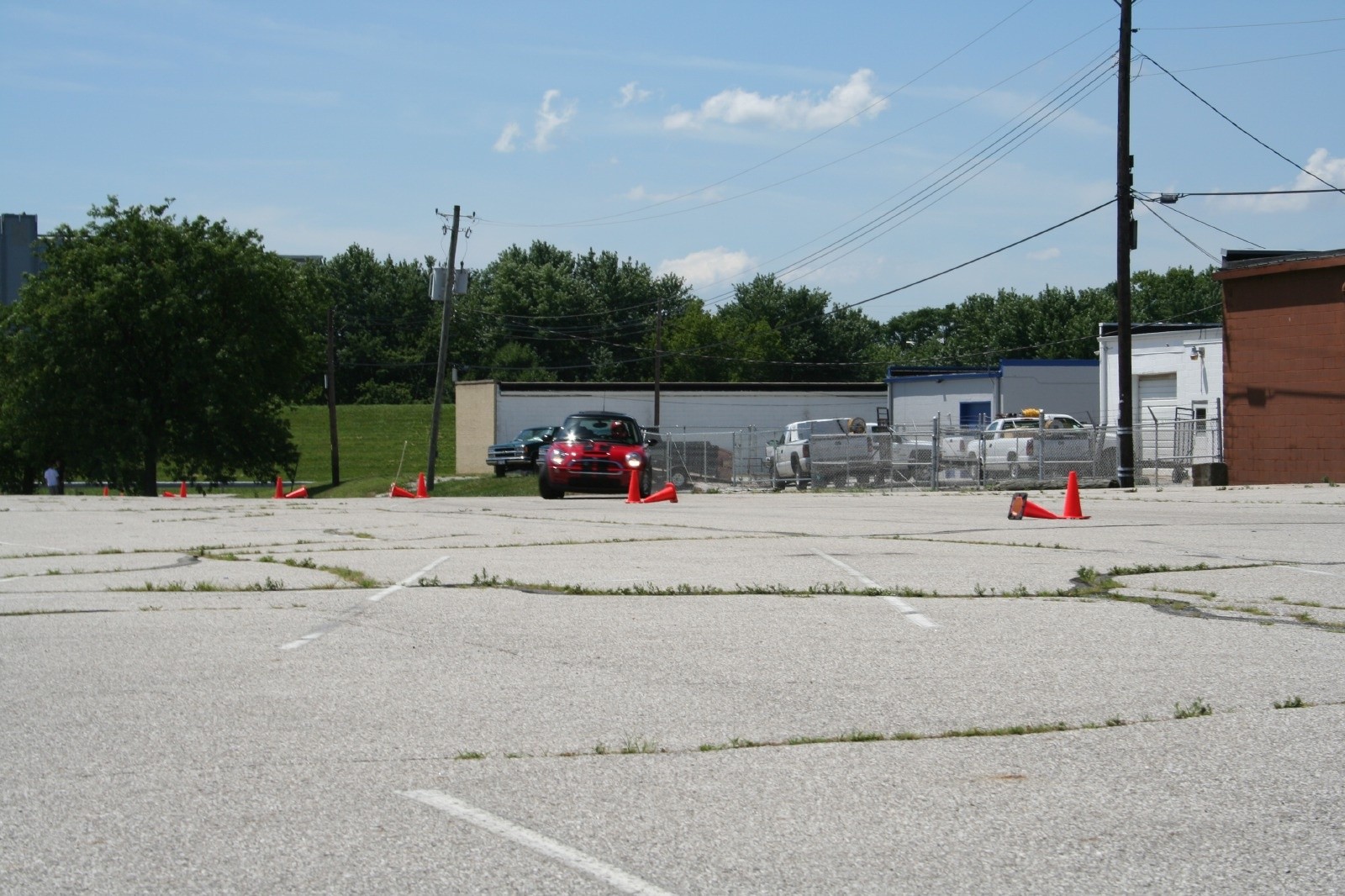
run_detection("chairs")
[573,426,588,438]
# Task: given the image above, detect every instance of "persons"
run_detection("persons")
[44,461,64,495]
[611,421,628,439]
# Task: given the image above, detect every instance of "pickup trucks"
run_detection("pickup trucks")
[766,422,945,481]
[768,417,875,494]
[635,426,719,489]
[942,413,1120,477]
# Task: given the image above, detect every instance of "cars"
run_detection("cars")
[485,425,559,478]
[539,411,659,498]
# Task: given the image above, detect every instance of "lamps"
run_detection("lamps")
[936,380,943,383]
[1189,344,1205,360]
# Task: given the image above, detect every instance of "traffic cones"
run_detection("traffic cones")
[162,491,176,497]
[625,469,644,502]
[639,483,679,504]
[1062,469,1092,520]
[102,485,109,495]
[1006,492,1066,521]
[389,483,414,497]
[415,472,431,498]
[284,484,309,499]
[179,478,188,498]
[283,476,288,498]
